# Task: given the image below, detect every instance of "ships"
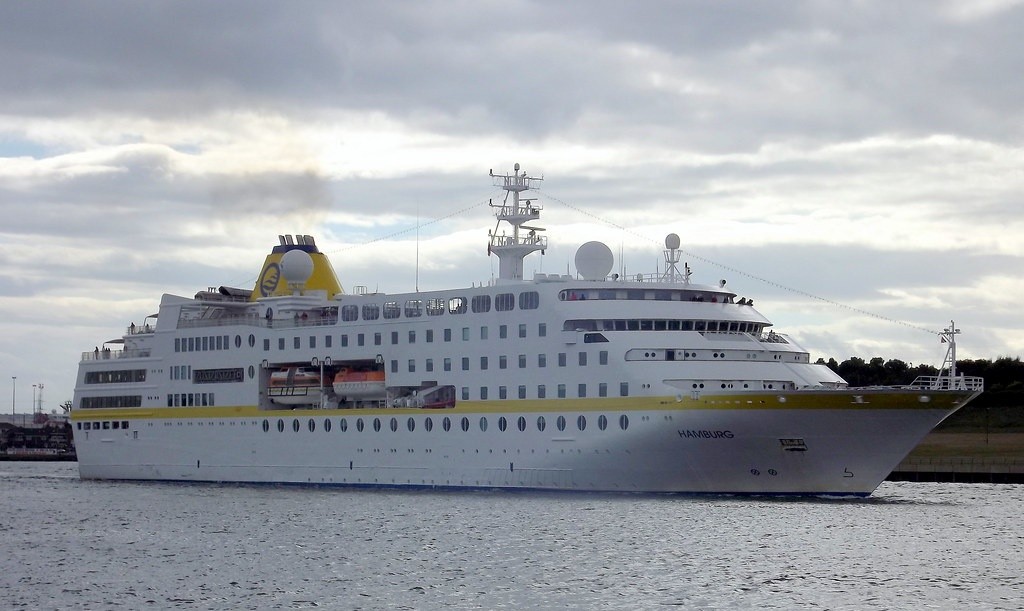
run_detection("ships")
[66,163,985,499]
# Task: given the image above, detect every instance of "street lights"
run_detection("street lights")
[32,385,36,426]
[11,376,17,426]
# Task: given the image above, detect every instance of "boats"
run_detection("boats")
[333,368,394,402]
[267,368,341,406]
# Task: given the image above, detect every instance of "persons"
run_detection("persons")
[579,294,584,300]
[294,312,308,327]
[266,314,272,328]
[569,292,576,300]
[692,295,754,306]
[94,346,99,360]
[768,329,775,339]
[130,322,135,335]
[145,324,149,330]
[456,303,467,313]
[102,346,110,359]
